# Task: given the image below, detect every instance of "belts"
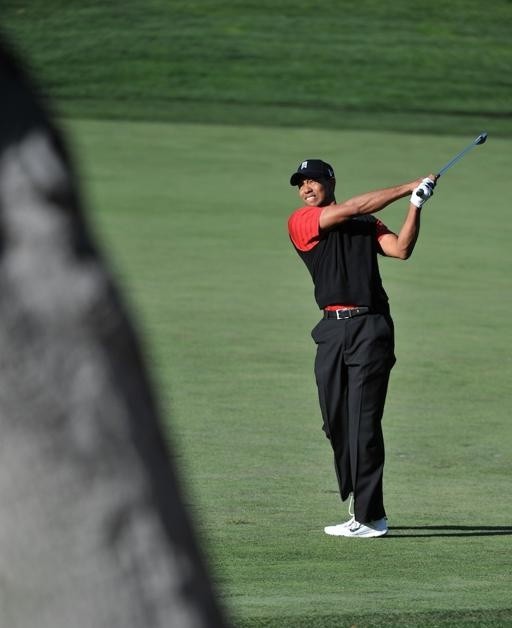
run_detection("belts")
[324,307,370,319]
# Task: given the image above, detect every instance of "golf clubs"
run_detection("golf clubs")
[416,134,488,195]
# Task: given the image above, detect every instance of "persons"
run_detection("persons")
[281,156,443,541]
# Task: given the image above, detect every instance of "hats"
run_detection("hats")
[289,159,336,184]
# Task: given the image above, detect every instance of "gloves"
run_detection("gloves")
[410,178,435,209]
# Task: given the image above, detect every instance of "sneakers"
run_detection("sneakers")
[323,516,388,538]
